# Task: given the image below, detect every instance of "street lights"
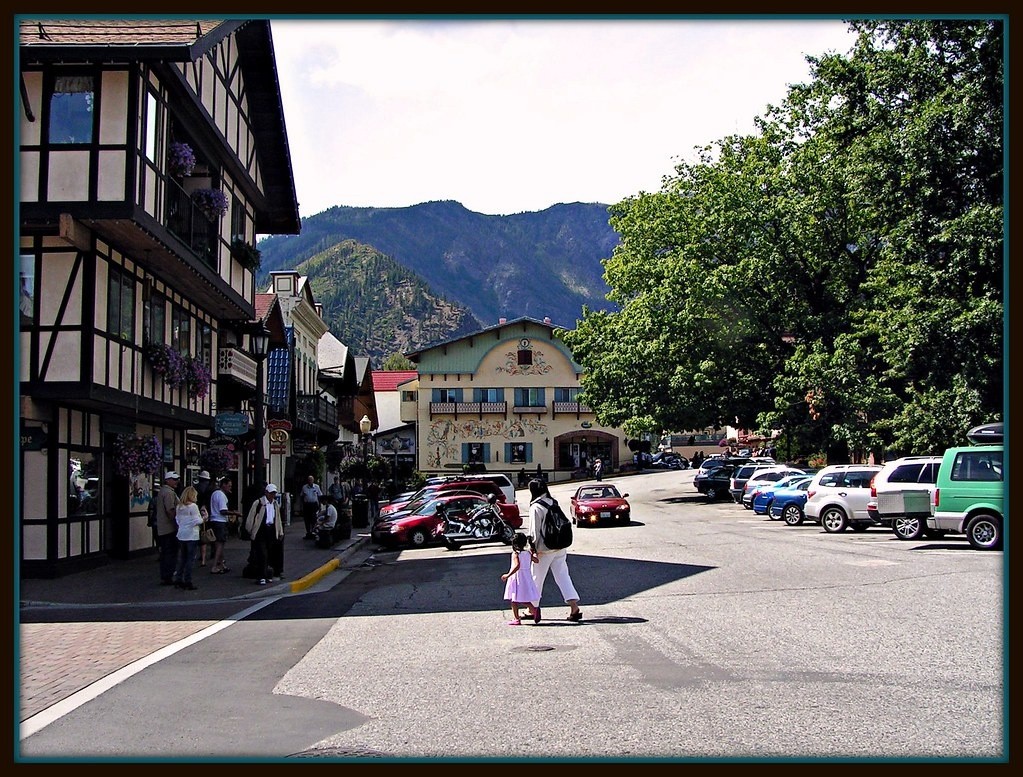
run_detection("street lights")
[391,437,401,490]
[471,448,477,471]
[359,414,371,501]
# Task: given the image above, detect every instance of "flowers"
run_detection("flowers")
[167,142,196,179]
[184,353,212,404]
[111,433,162,484]
[199,447,234,477]
[340,453,393,481]
[146,342,188,391]
[189,187,229,223]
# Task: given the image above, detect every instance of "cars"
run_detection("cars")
[633,450,690,470]
[693,452,816,526]
[370,471,524,547]
[569,484,631,528]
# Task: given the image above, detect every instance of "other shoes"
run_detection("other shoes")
[534,607,541,623]
[258,578,266,584]
[303,532,310,539]
[508,620,521,625]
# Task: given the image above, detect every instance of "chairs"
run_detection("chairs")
[582,494,593,499]
[603,492,613,498]
[862,479,870,488]
[851,479,861,488]
[978,461,988,480]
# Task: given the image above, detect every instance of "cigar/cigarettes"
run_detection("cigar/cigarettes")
[228,490,232,493]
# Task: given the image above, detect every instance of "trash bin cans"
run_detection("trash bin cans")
[351,494,369,528]
[281,493,290,527]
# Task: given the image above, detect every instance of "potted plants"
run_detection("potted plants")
[233,238,263,273]
[305,450,327,491]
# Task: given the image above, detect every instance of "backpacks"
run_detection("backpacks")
[535,497,573,549]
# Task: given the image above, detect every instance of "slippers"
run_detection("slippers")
[211,568,225,574]
[520,612,534,619]
[567,612,582,620]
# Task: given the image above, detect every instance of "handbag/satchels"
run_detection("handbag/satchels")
[241,517,250,541]
[201,528,216,543]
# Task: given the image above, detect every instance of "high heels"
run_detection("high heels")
[183,582,198,590]
[174,579,183,588]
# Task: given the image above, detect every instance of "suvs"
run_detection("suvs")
[926,422,1005,550]
[866,455,1001,540]
[803,463,889,534]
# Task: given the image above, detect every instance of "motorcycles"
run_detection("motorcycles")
[435,494,514,552]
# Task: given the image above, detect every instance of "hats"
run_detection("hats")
[198,471,211,480]
[153,485,162,492]
[165,471,180,479]
[266,484,278,492]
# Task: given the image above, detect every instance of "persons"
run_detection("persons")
[312,496,338,544]
[723,448,729,458]
[210,478,242,575]
[600,488,613,498]
[300,476,323,540]
[245,483,284,585]
[330,474,345,504]
[197,469,210,567]
[501,533,539,626]
[520,478,583,622]
[748,445,777,461]
[594,459,603,481]
[156,471,181,585]
[174,487,204,590]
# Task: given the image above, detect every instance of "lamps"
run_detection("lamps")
[581,436,588,443]
[310,435,319,452]
[545,437,550,448]
[624,438,629,447]
